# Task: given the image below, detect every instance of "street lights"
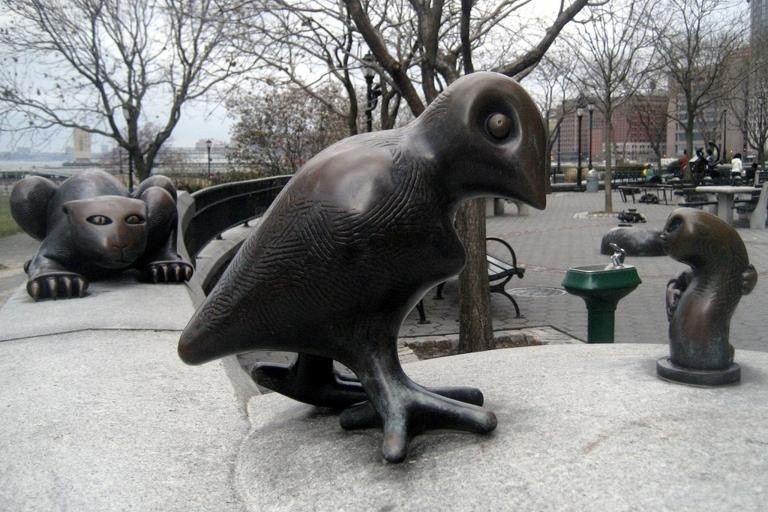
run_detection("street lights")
[586,100,598,175]
[573,104,587,193]
[206,137,213,179]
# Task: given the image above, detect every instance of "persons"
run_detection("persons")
[743,163,757,180]
[642,163,663,184]
[730,152,742,182]
[678,145,717,184]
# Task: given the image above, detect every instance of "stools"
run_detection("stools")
[483,236,525,320]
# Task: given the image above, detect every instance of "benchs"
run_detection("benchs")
[619,167,768,232]
